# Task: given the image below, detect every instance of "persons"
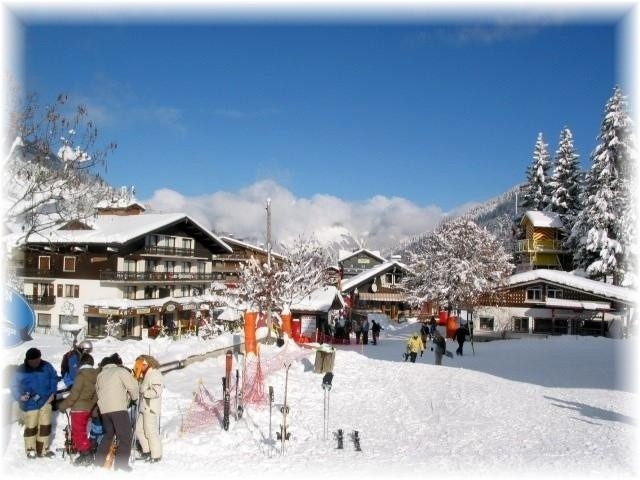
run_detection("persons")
[407,331,424,362]
[429,315,437,330]
[354,319,384,345]
[453,323,470,356]
[432,331,446,365]
[10,341,162,472]
[420,321,431,350]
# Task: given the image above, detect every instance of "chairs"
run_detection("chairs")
[25,445,163,473]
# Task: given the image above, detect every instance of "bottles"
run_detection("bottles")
[25,348,42,359]
[80,354,122,365]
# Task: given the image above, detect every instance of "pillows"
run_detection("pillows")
[79,340,93,353]
